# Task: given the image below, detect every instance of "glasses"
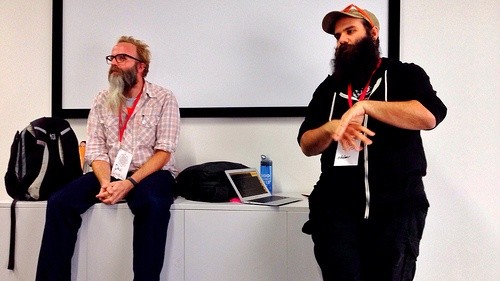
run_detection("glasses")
[106,54,143,64]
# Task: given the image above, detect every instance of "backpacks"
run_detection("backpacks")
[5,117,83,201]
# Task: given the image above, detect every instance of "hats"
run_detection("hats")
[322,3,379,34]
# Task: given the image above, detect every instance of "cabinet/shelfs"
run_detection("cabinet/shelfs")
[0,193,323,281]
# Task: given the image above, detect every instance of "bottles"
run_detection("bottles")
[259,154,272,196]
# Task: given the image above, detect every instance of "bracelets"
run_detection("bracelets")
[128,176,139,187]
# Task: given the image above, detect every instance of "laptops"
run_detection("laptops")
[225,167,302,206]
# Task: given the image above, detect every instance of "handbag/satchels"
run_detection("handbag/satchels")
[176,161,250,202]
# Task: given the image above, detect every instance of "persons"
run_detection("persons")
[36,37,181,281]
[297,4,447,281]
